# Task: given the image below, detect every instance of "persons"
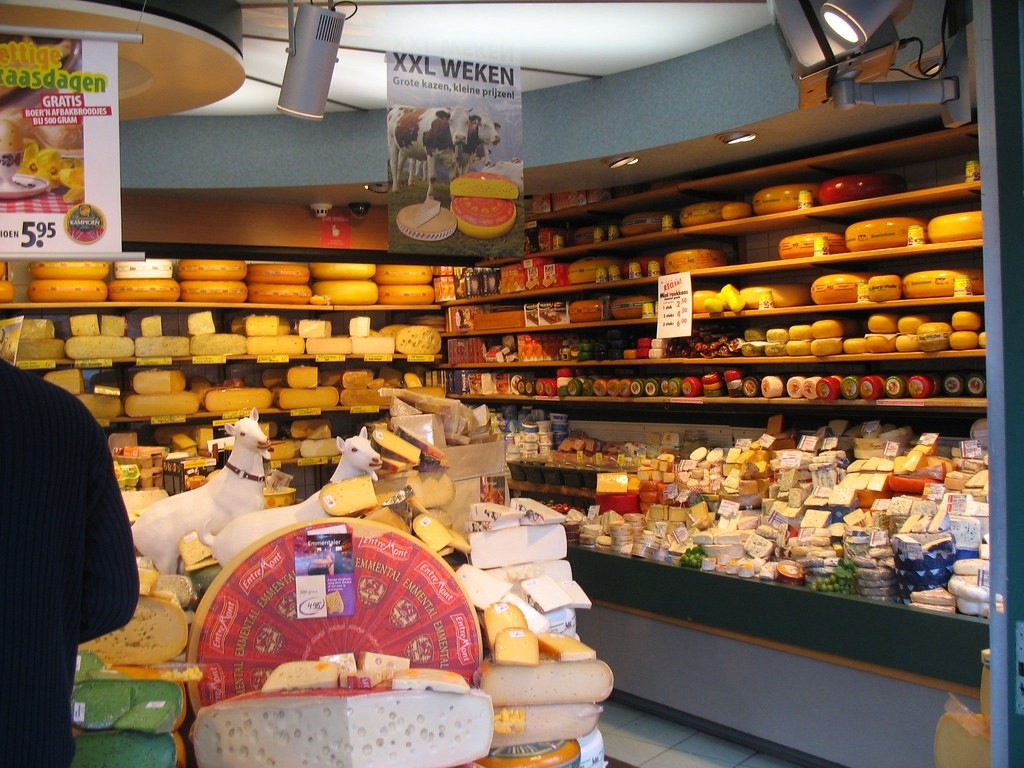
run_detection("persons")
[0,356,140,768]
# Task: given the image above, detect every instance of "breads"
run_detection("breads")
[396,170,520,241]
[0,312,615,748]
[554,411,988,617]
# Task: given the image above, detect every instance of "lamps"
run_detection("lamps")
[819,0,901,46]
[277,4,346,123]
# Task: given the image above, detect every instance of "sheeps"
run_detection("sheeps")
[200,426,384,571]
[121,406,273,578]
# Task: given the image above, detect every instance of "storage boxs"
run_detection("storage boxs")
[426,188,610,396]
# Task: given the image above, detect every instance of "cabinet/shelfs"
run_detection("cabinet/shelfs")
[436,123,988,407]
[0,302,442,467]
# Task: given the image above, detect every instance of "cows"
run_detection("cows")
[387,104,504,204]
